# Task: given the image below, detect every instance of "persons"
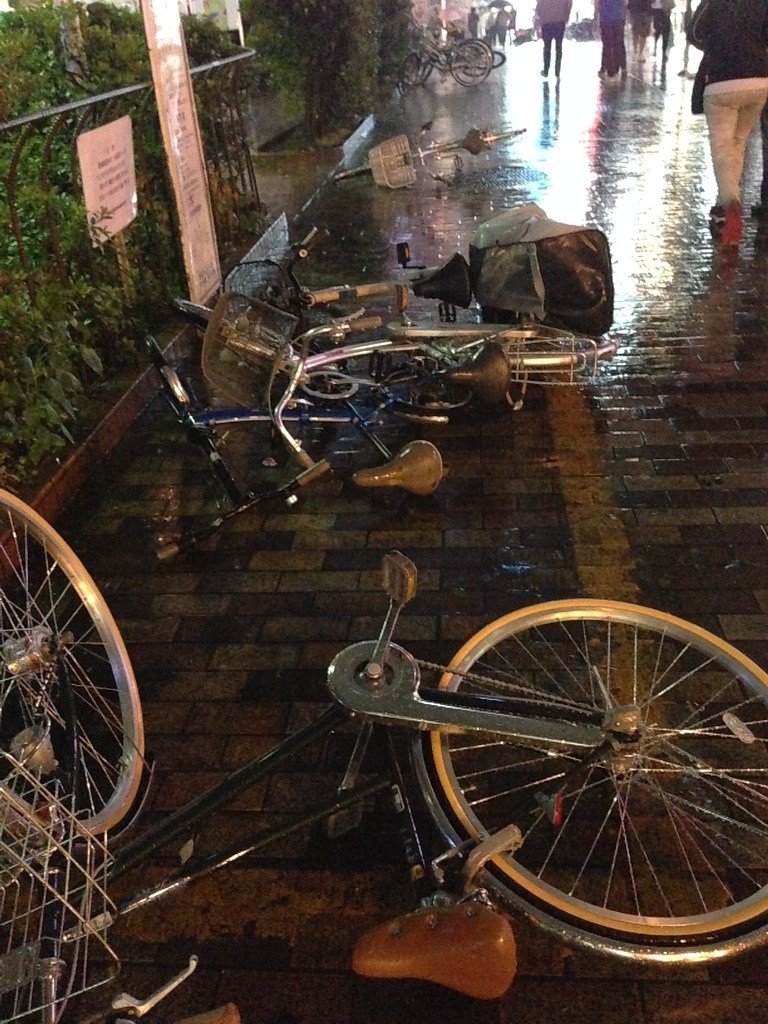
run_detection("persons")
[588,0,679,81]
[750,97,768,222]
[683,0,768,245]
[534,0,573,78]
[468,6,521,52]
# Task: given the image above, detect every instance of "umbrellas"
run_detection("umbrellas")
[486,0,513,10]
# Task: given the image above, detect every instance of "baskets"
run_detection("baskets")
[223,260,299,317]
[369,135,416,188]
[201,290,298,413]
[0,746,121,1024]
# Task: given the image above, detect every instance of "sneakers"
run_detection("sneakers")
[710,201,744,246]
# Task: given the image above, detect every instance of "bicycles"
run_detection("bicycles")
[394,0,506,99]
[2,480,766,1024]
[331,116,529,190]
[104,207,622,575]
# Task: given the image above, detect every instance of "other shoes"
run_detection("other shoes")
[540,50,688,81]
